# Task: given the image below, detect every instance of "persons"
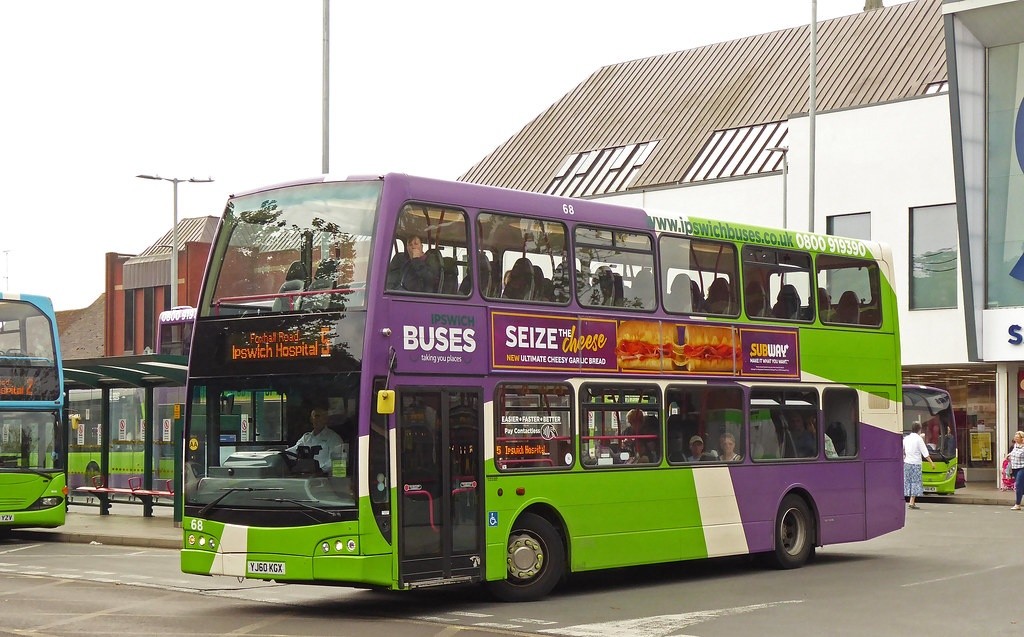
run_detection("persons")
[562,409,839,465]
[359,235,434,308]
[281,408,344,479]
[903,420,955,510]
[1006,431,1024,509]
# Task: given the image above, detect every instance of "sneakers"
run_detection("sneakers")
[1011,504,1021,510]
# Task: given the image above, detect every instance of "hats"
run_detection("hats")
[689,435,703,447]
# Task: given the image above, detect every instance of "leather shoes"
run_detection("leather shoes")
[908,503,919,509]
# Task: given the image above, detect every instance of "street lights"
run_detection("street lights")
[135,172,216,356]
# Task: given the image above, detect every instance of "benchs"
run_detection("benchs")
[76,475,174,517]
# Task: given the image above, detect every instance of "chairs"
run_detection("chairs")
[825,421,847,456]
[272,258,337,312]
[385,252,860,325]
[643,413,698,462]
[328,412,353,443]
[0,456,18,467]
[0,348,28,357]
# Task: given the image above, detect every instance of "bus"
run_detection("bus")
[902,382,959,497]
[1,385,287,495]
[156,305,198,357]
[178,169,908,605]
[0,292,82,543]
